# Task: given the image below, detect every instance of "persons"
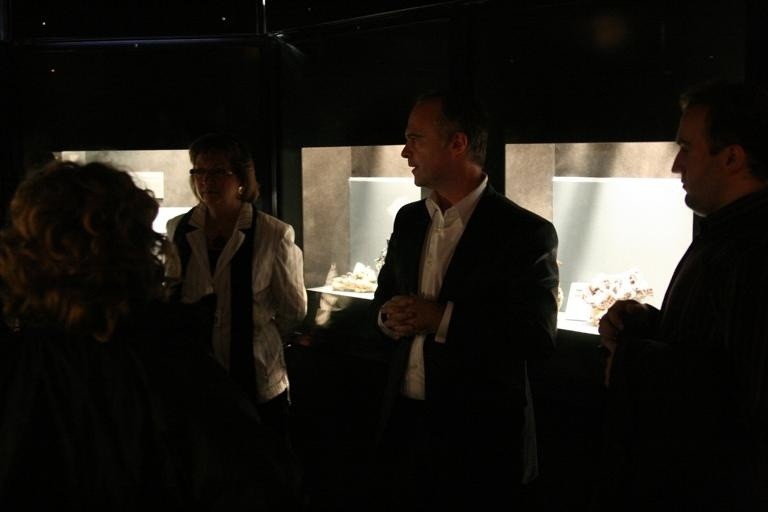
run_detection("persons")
[598,75,768,510]
[362,83,557,509]
[0,158,305,509]
[162,132,311,508]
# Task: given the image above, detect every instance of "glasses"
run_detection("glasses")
[189,167,235,177]
